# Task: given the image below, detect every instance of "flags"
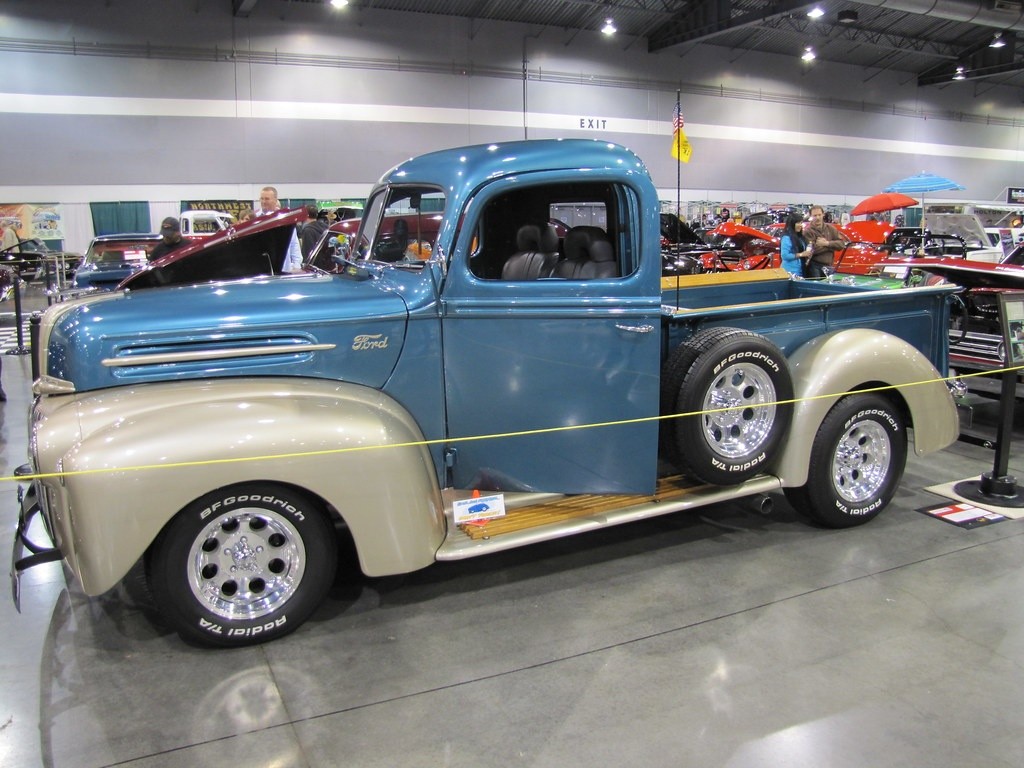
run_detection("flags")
[671,103,692,165]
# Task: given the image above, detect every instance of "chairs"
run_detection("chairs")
[500,218,563,279]
[549,225,622,279]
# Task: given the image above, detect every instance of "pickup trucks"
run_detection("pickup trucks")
[24,138,962,652]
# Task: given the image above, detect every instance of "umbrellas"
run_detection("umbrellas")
[850,170,964,256]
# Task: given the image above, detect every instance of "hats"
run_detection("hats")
[159,217,180,237]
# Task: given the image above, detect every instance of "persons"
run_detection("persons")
[1,221,20,254]
[780,211,811,276]
[146,217,193,264]
[803,207,846,278]
[236,186,355,264]
[691,208,735,229]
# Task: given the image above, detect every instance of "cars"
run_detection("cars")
[176,209,237,238]
[665,208,1024,380]
[72,232,165,287]
[289,210,445,277]
